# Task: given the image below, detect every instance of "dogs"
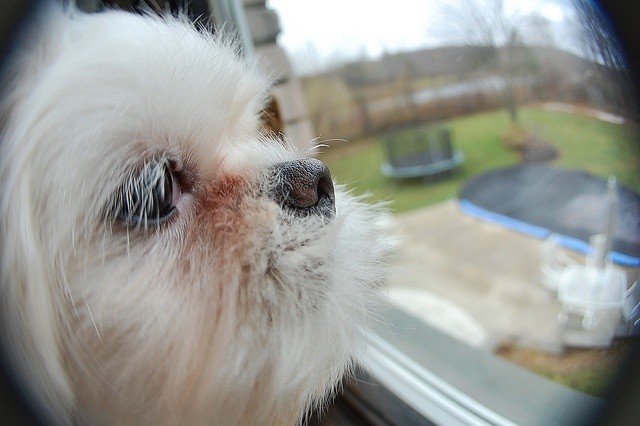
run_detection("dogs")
[0,0,372,424]
